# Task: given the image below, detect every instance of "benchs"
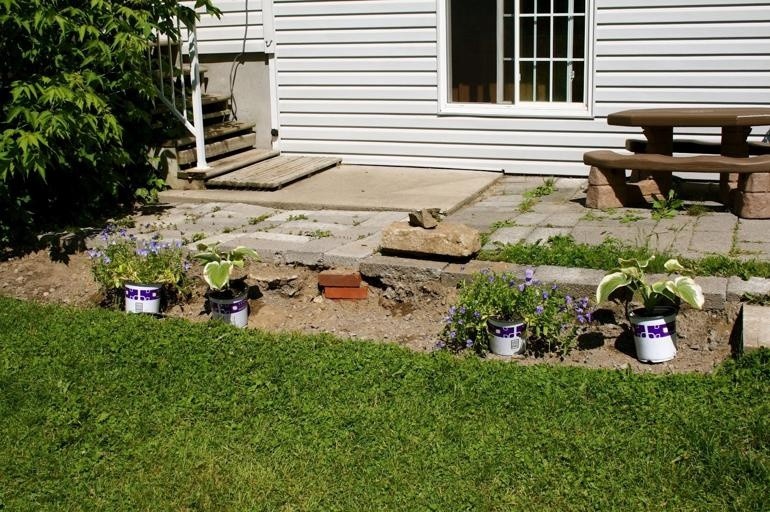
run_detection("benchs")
[581,150,770,219]
[626,139,770,195]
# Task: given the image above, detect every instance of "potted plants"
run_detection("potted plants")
[596,253,704,363]
[189,242,263,328]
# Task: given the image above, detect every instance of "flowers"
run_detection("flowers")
[89,225,189,285]
[433,266,595,356]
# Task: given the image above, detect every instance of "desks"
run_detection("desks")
[607,108,770,203]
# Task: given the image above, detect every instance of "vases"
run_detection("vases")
[486,315,528,357]
[125,282,163,314]
